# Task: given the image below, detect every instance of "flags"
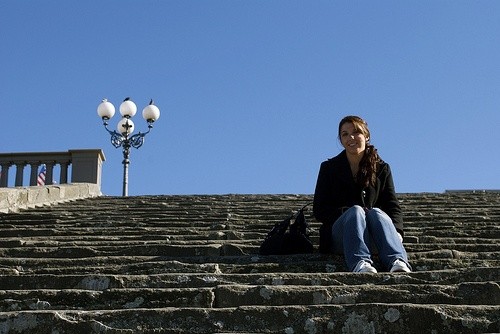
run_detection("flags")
[36,164,55,186]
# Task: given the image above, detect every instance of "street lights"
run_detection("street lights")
[97,97,161,196]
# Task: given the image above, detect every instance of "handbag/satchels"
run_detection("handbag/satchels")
[260,202,318,256]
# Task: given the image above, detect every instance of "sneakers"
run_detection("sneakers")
[353,259,377,272]
[389,259,410,271]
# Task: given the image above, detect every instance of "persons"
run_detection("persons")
[312,115,413,274]
